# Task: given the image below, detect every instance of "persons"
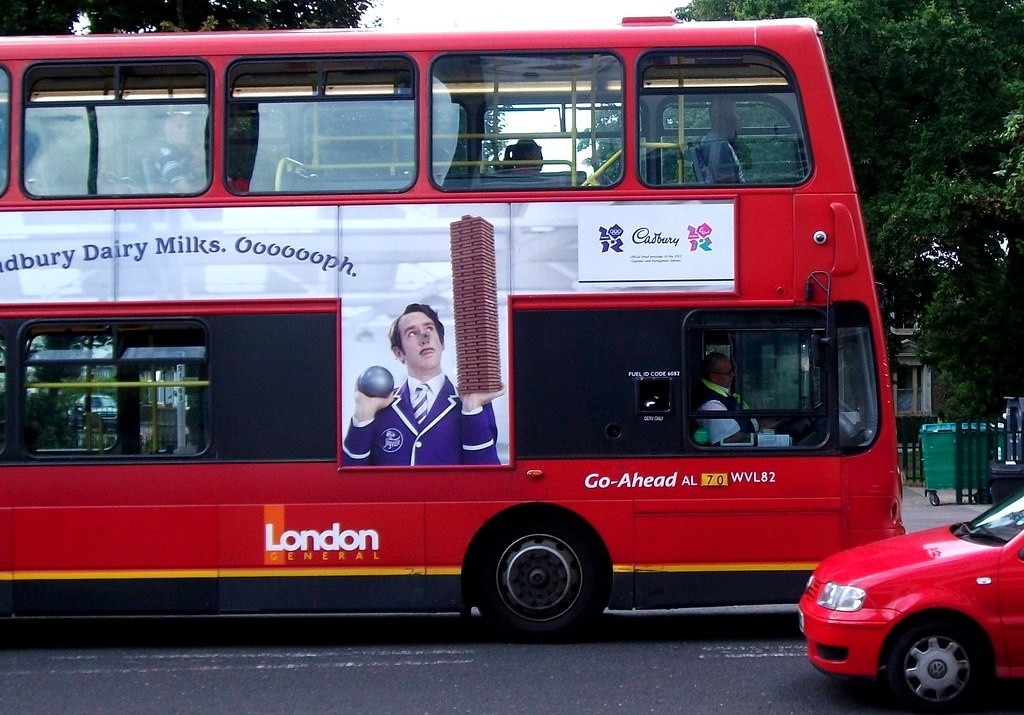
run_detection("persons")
[155,113,206,193]
[503,138,543,173]
[694,353,791,443]
[700,96,746,183]
[342,304,506,467]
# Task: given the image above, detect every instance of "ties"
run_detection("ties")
[412,384,429,424]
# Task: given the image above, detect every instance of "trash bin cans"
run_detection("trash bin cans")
[919,421,993,505]
[989,421,1021,462]
[156,407,177,450]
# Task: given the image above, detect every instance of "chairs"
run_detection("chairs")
[0,137,747,202]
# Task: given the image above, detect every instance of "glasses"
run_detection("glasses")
[711,369,732,376]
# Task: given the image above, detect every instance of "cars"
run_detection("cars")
[796,485,1024,715]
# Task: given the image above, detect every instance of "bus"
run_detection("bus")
[0,12,905,665]
